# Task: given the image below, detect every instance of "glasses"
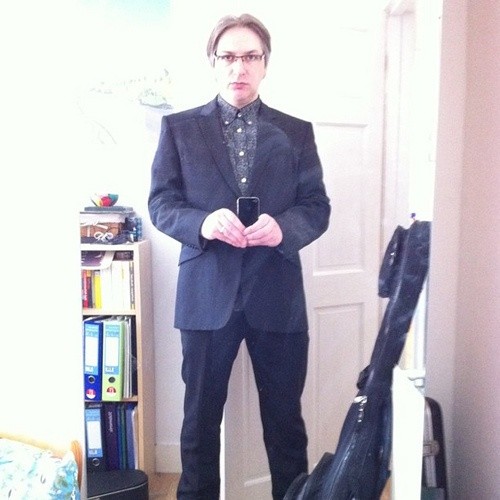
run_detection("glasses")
[214,50,265,67]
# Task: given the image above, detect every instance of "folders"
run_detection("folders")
[102,402,118,470]
[83,315,103,402]
[100,316,124,403]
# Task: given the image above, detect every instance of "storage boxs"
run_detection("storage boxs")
[80,207,135,224]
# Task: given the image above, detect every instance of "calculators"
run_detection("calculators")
[85,404,105,473]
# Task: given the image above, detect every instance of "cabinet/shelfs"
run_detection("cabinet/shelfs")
[81,238,155,489]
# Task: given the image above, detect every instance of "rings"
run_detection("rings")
[219,226,225,233]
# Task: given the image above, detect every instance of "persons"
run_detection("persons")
[147,13,331,500]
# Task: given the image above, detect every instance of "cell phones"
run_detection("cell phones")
[236,197,260,228]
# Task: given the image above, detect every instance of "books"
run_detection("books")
[80,250,139,469]
